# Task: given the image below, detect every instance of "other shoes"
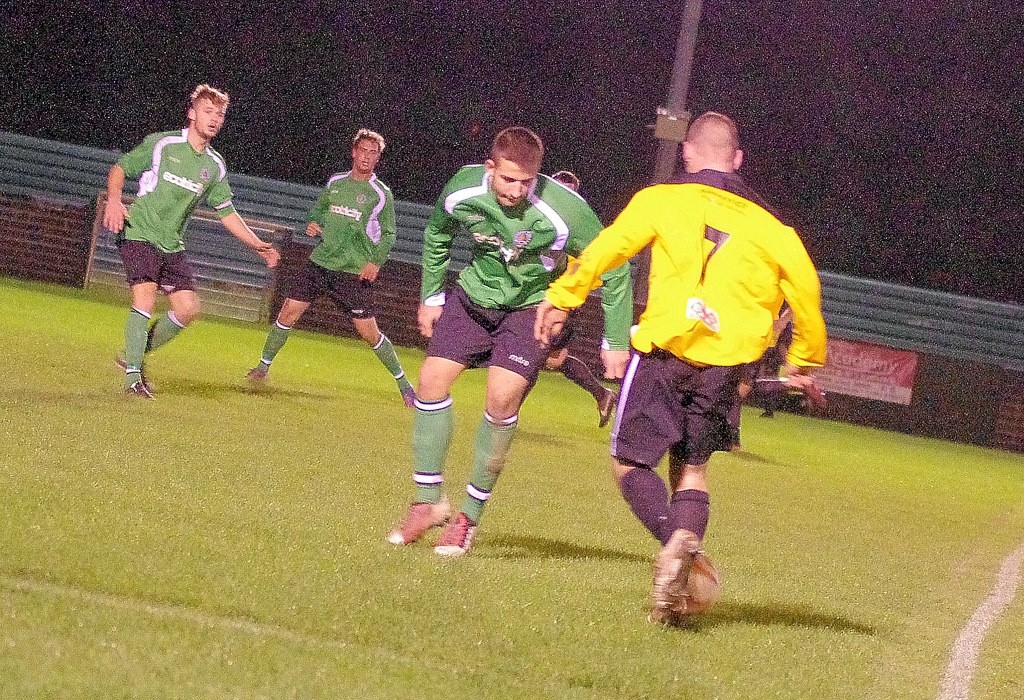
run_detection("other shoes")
[761,411,777,420]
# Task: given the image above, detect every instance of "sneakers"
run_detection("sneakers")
[244,364,269,383]
[387,491,453,548]
[596,384,617,428]
[398,385,417,409]
[654,524,702,614]
[801,373,830,410]
[434,513,478,559]
[116,357,141,373]
[125,379,155,403]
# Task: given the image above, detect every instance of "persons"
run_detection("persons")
[248,129,419,411]
[548,170,620,428]
[103,85,282,408]
[531,113,829,626]
[736,300,828,417]
[387,128,634,557]
